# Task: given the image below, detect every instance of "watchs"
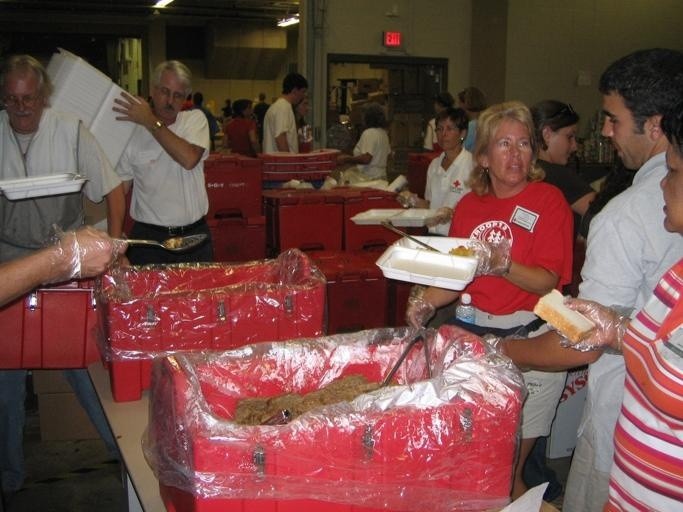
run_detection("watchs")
[149,119,164,133]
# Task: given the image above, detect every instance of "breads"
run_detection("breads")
[533,288,596,345]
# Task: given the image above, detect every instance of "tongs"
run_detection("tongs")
[381,334,433,388]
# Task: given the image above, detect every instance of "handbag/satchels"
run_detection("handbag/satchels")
[449,318,528,350]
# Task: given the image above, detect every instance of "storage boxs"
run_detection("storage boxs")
[1,146,527,512]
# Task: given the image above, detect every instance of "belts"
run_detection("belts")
[133,216,207,238]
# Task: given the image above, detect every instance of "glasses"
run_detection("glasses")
[1,91,43,108]
[539,101,576,124]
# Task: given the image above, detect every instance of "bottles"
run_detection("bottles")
[455,293,475,334]
[574,110,615,169]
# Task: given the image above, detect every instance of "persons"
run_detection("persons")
[1,224,129,308]
[1,53,126,496]
[335,104,395,180]
[180,72,326,190]
[394,48,683,512]
[111,58,215,265]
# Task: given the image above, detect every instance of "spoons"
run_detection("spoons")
[114,232,208,253]
